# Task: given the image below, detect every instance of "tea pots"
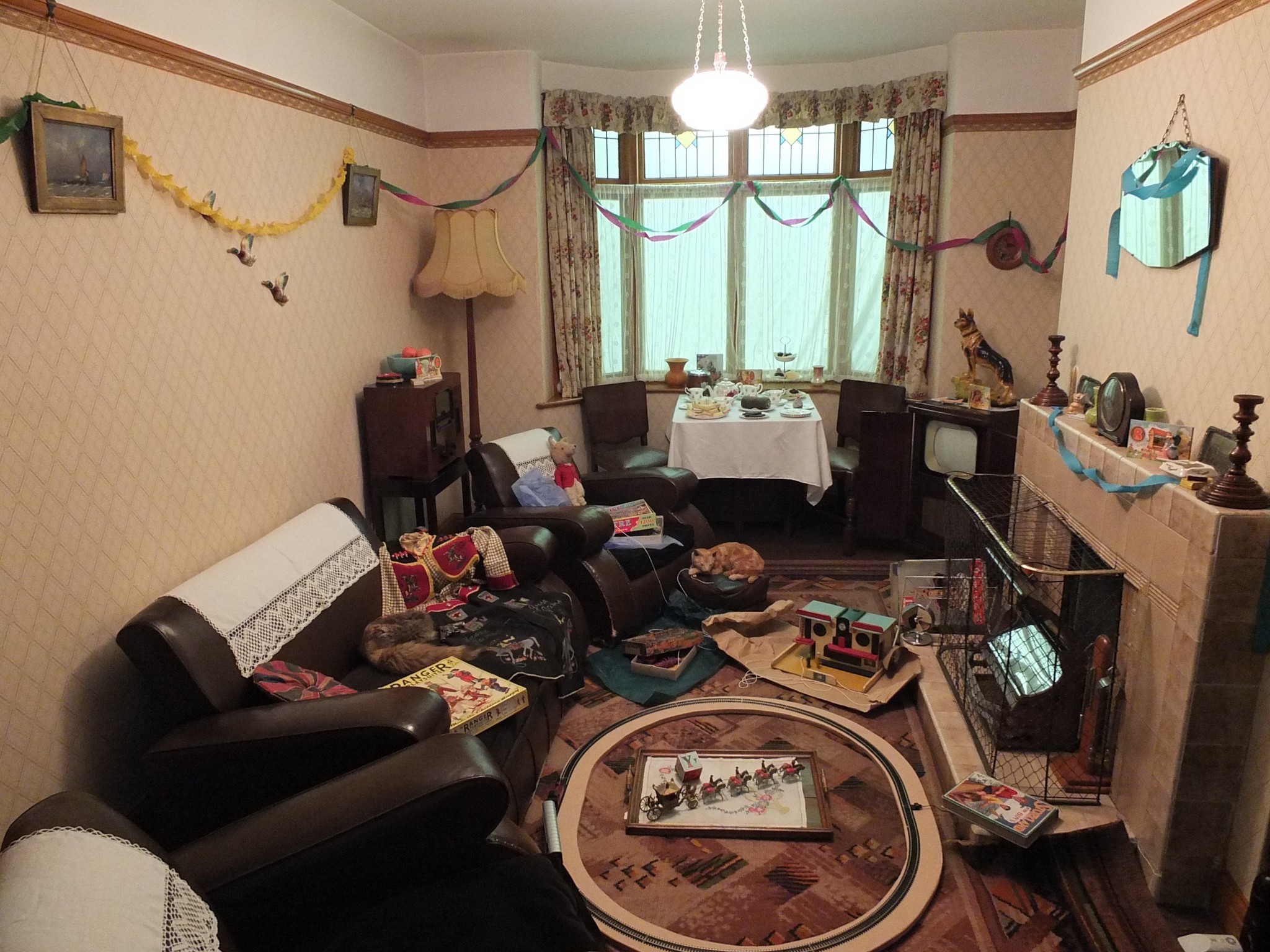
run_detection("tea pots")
[707,378,743,400]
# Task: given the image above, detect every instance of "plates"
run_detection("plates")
[782,392,806,401]
[784,403,814,410]
[766,397,788,406]
[738,404,776,411]
[678,403,693,410]
[686,410,729,420]
[739,411,770,419]
[779,409,811,418]
[682,396,709,402]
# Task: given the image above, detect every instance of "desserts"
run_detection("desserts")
[741,396,771,410]
[686,398,731,418]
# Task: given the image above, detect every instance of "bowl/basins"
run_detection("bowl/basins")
[386,352,437,374]
[713,396,734,408]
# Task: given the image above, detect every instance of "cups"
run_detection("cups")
[765,389,786,401]
[739,383,763,398]
[685,387,704,400]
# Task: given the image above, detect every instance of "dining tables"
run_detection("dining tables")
[668,386,833,535]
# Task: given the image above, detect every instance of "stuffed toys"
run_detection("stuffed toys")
[549,435,587,507]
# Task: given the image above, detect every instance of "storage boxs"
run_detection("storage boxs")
[622,626,705,678]
[378,654,530,738]
[604,498,666,543]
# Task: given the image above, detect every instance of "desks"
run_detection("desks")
[368,451,472,544]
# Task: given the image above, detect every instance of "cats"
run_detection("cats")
[361,610,481,673]
[688,542,765,584]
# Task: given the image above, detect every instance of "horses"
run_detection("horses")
[698,762,806,805]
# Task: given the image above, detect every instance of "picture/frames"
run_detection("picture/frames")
[28,101,127,216]
[628,744,835,841]
[344,164,382,228]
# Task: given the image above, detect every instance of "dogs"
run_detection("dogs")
[954,308,1014,399]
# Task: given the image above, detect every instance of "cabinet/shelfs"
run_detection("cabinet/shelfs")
[903,387,1021,565]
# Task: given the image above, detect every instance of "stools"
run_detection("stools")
[678,564,770,617]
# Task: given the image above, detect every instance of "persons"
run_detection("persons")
[709,757,798,786]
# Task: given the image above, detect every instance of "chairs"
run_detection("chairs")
[582,379,669,478]
[828,378,905,555]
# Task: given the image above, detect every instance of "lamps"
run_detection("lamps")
[412,204,531,526]
[670,0,770,134]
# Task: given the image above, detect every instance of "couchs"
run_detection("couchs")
[2,784,615,951]
[118,492,590,833]
[465,423,716,645]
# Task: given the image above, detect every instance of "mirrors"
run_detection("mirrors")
[1117,139,1217,271]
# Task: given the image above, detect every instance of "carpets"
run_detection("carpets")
[519,552,1150,952]
